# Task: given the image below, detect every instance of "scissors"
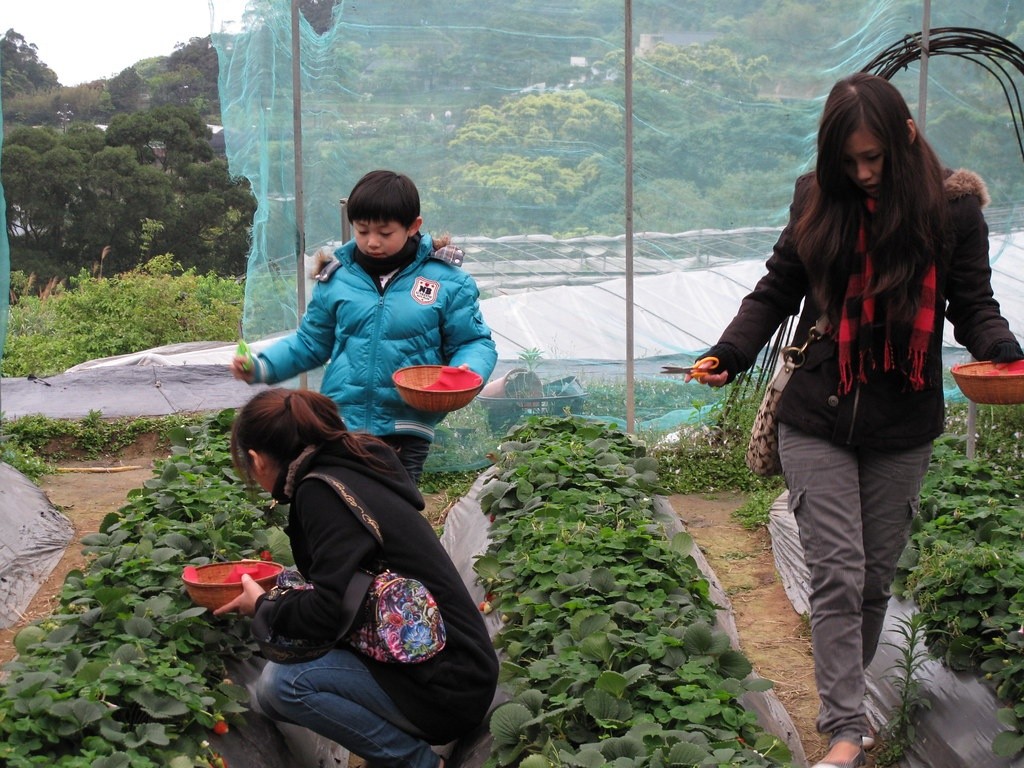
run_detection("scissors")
[236,318,252,370]
[660,357,718,386]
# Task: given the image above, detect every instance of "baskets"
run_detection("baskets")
[182,560,285,610]
[392,364,483,413]
[949,359,1024,405]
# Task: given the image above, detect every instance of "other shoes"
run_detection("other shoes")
[811,739,867,768]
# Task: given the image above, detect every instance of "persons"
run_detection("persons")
[684,72,1024,768]
[236,170,498,489]
[213,388,501,768]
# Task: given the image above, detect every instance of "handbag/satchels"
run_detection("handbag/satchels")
[337,567,448,666]
[744,346,806,477]
